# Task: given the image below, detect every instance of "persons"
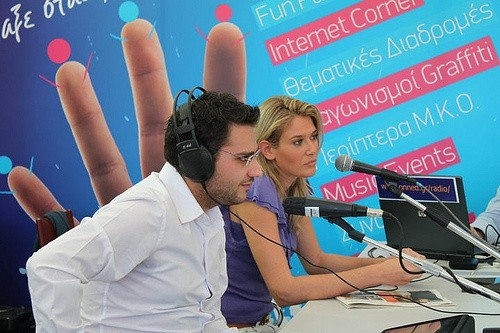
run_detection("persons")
[470,186,500,246]
[219,96,427,332]
[7,18,246,227]
[26,91,262,333]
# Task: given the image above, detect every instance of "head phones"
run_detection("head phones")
[172,86,215,183]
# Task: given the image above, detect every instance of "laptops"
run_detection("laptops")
[375,174,496,263]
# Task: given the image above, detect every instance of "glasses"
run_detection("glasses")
[215,150,261,166]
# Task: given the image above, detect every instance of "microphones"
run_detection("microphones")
[283,197,393,220]
[335,154,418,185]
